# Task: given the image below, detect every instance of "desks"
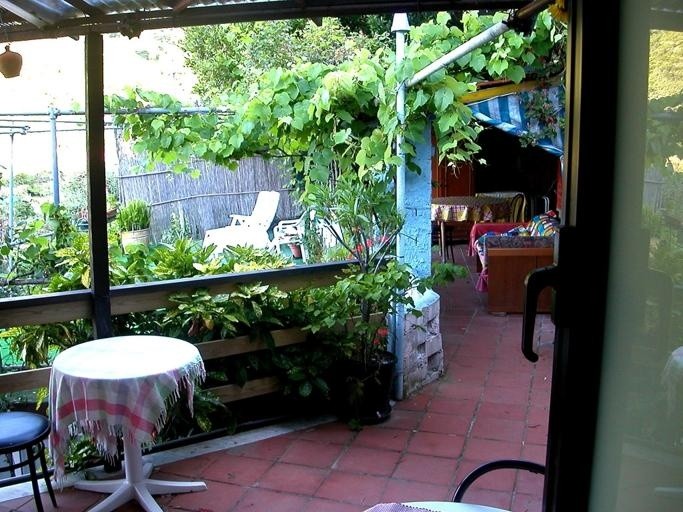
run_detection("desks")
[429,195,510,264]
[49,335,206,510]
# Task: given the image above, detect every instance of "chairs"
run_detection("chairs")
[203,190,316,264]
[468,214,559,316]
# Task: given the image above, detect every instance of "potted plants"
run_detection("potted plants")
[118,201,150,255]
[306,170,406,425]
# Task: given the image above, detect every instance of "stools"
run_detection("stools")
[0,412,60,511]
